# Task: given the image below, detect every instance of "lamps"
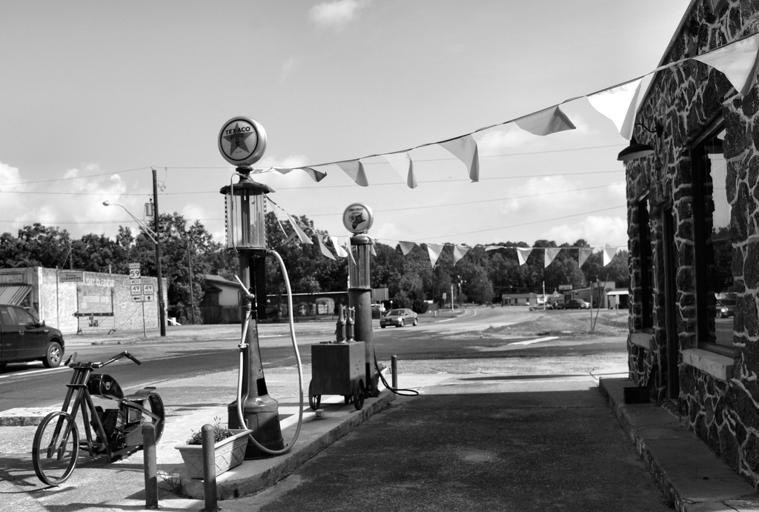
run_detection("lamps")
[614,119,666,162]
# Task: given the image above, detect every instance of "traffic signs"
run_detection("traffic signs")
[144,285,153,294]
[129,262,141,270]
[130,279,141,285]
[131,285,141,295]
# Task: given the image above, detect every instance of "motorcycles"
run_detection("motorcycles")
[30,350,168,485]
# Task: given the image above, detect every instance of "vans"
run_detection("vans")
[371,302,385,313]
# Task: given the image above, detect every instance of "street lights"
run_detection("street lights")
[104,200,168,339]
[457,274,467,307]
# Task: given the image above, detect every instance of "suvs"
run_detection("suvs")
[554,298,565,308]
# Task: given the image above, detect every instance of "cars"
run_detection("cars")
[715,297,737,319]
[0,302,66,370]
[381,308,419,329]
[564,297,591,309]
[528,302,554,311]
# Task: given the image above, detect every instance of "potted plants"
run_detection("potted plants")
[173,415,253,481]
